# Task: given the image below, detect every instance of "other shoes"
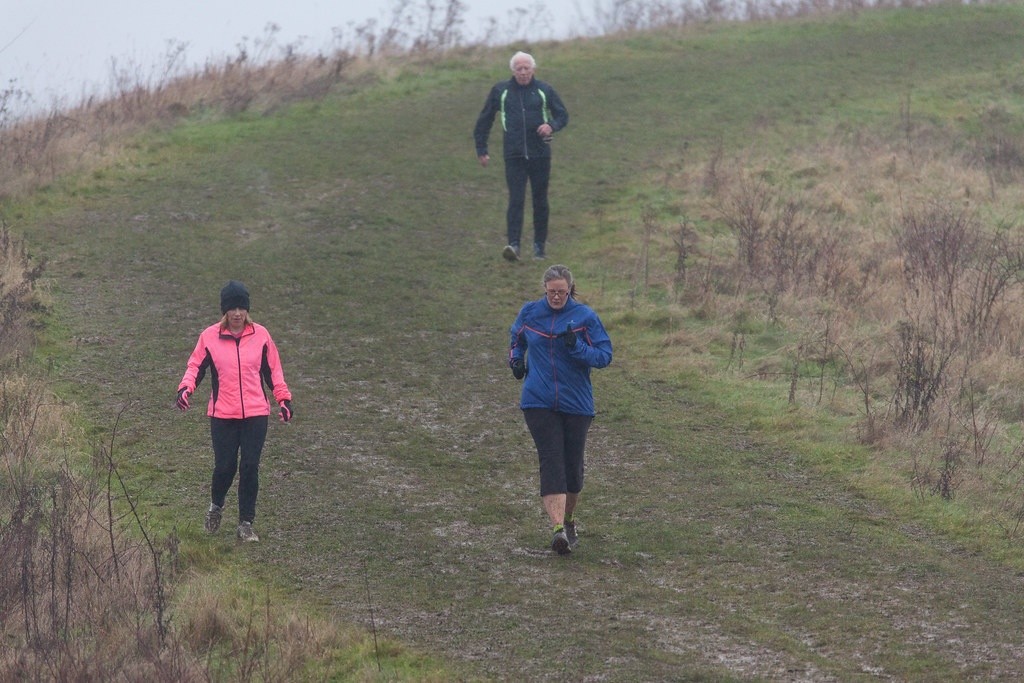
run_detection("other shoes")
[533,250,545,262]
[503,244,521,260]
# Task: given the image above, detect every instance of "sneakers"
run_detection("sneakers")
[236,521,259,543]
[563,514,578,547]
[204,503,223,534]
[551,529,572,555]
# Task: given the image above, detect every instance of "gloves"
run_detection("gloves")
[556,324,576,348]
[512,361,524,380]
[278,399,293,423]
[176,388,191,411]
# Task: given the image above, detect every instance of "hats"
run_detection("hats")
[220,280,249,314]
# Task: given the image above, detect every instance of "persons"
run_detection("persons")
[510,264,612,553]
[176,284,293,543]
[474,52,569,261]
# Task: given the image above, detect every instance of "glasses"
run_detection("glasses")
[544,289,571,297]
